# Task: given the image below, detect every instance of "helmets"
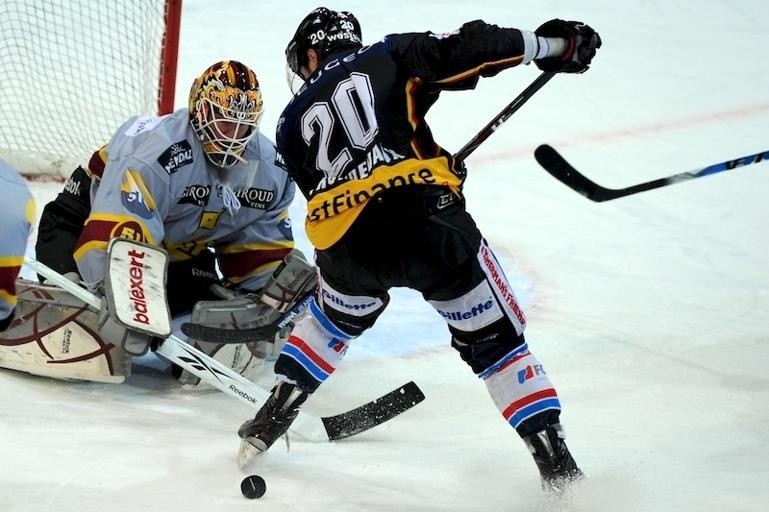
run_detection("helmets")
[188,60,263,170]
[285,7,363,95]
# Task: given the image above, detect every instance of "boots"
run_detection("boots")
[532,427,581,497]
[238,382,308,452]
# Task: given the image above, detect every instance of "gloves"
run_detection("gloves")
[535,19,601,74]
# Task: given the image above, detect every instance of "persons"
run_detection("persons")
[233,8,605,491]
[0,61,317,388]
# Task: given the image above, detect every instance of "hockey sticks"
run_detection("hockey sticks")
[180,63,563,342]
[534,142,769,202]
[24,256,426,444]
